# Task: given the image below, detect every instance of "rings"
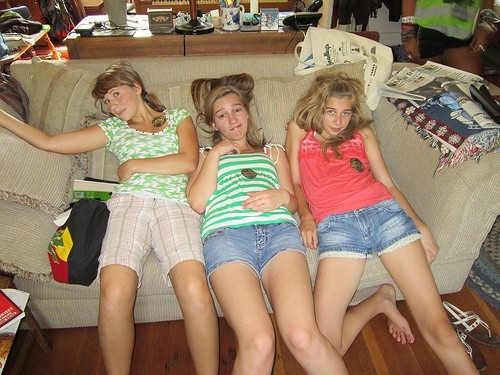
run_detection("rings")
[408,55,413,59]
[479,45,485,52]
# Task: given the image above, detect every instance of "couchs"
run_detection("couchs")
[0,53,500,329]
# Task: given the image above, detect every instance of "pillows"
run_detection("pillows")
[0,55,374,283]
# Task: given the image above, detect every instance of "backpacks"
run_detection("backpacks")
[44,7,75,44]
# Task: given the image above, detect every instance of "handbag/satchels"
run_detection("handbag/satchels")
[294,26,394,111]
[47,199,110,286]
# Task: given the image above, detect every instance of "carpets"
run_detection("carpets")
[466,214,500,310]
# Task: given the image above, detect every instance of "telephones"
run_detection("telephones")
[282,0,323,31]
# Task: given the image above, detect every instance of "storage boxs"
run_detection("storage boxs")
[147,7,173,29]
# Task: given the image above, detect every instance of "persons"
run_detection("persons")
[185,72,349,375]
[285,72,480,375]
[401,0,495,77]
[0,61,220,375]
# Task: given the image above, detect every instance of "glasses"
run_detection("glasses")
[104,20,136,30]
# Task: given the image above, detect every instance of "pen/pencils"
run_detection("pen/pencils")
[220,0,240,8]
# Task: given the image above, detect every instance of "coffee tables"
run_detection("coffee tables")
[1,277,52,375]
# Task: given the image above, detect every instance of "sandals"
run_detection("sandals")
[455,329,487,372]
[443,300,500,349]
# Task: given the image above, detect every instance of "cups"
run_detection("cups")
[219,7,241,31]
[103,0,127,25]
[211,10,222,28]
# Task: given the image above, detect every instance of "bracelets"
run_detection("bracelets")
[401,16,417,43]
[477,8,499,35]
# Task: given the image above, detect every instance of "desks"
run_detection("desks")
[63,14,307,62]
[0,25,60,76]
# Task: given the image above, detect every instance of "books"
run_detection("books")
[0,289,26,332]
